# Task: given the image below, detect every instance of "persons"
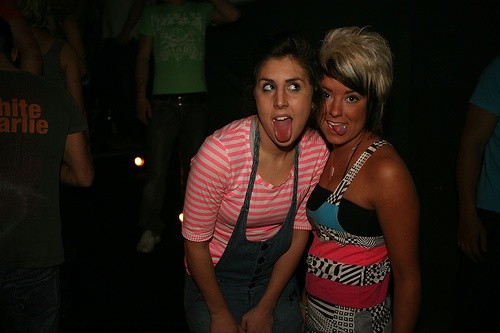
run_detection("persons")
[0,0,242,333]
[181,27,333,333]
[456,58,500,333]
[301,26,423,333]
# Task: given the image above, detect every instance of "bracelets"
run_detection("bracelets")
[301,301,309,310]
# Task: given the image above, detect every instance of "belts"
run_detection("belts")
[153,94,209,105]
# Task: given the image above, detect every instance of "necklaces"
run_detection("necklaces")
[328,145,357,181]
